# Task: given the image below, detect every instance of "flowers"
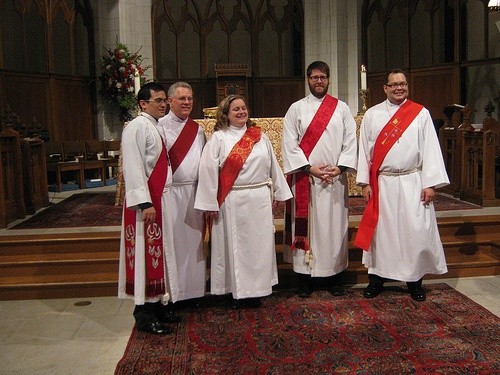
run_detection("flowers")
[87,40,161,117]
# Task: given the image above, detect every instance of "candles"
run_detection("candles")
[361,65,367,91]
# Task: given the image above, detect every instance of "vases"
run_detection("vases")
[118,106,134,121]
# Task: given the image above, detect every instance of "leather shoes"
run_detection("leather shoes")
[156,302,181,323]
[408,284,426,301]
[363,281,385,297]
[139,319,170,334]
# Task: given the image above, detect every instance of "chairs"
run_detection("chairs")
[47,141,120,193]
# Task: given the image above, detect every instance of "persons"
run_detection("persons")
[193,94,295,304]
[356,69,449,300]
[120,81,206,335]
[283,61,357,298]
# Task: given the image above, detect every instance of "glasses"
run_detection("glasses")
[309,75,328,82]
[386,81,408,89]
[145,98,170,104]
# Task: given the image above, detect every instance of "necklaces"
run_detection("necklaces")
[386,99,400,143]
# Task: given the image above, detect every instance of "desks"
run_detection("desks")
[191,116,364,197]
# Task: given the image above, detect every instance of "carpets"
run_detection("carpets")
[7,192,483,230]
[113,283,500,375]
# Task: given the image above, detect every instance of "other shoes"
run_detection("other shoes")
[327,281,345,296]
[300,288,313,297]
[215,297,233,315]
